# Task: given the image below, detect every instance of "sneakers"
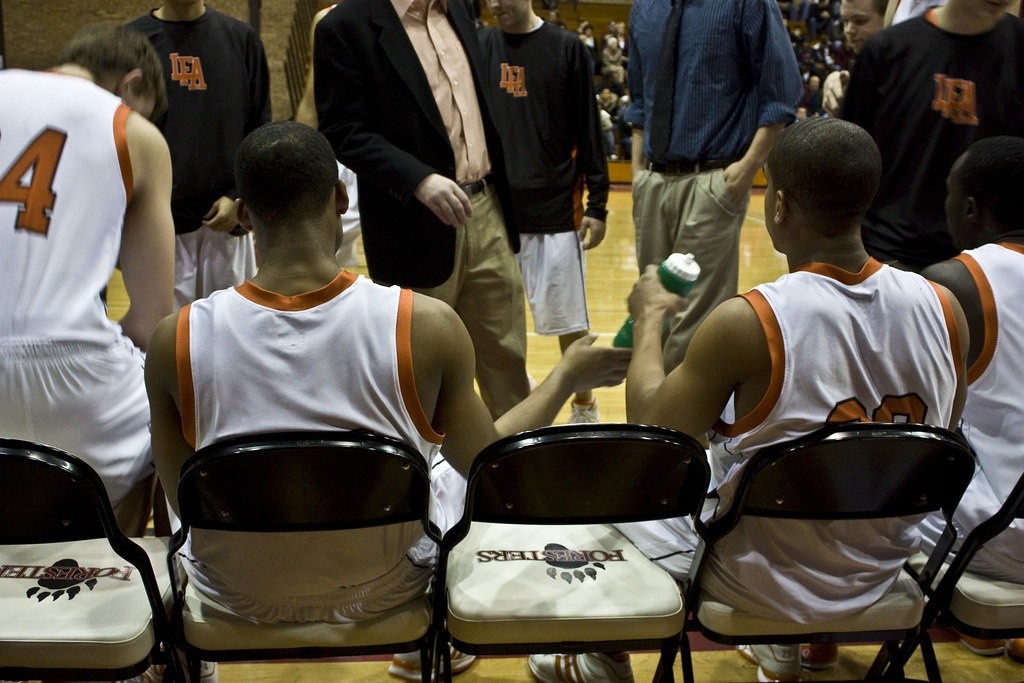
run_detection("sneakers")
[146,641,219,683]
[800,643,839,670]
[952,626,1024,662]
[738,642,802,683]
[567,398,600,425]
[528,652,635,683]
[387,642,476,682]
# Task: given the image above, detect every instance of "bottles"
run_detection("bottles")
[612,252,701,349]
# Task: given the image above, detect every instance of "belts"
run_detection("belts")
[646,158,737,177]
[460,172,493,197]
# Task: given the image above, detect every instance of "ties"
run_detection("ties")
[649,0,687,155]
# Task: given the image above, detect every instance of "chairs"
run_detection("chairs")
[0,423,1024,683]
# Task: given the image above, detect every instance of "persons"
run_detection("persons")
[529,117,970,683]
[122,0,247,313]
[0,24,176,538]
[542,0,634,162]
[622,0,805,377]
[147,120,633,683]
[778,0,856,125]
[478,0,611,424]
[822,0,1024,275]
[295,0,533,421]
[919,136,1024,656]
